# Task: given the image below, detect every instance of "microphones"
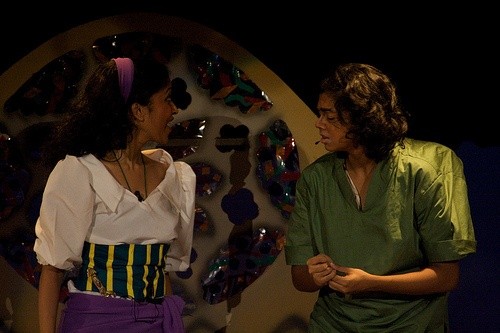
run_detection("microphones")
[315,141,320,145]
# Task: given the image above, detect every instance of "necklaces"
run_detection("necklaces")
[113,149,148,201]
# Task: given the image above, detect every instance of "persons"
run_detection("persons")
[32,57,196,333]
[284,62,477,333]
